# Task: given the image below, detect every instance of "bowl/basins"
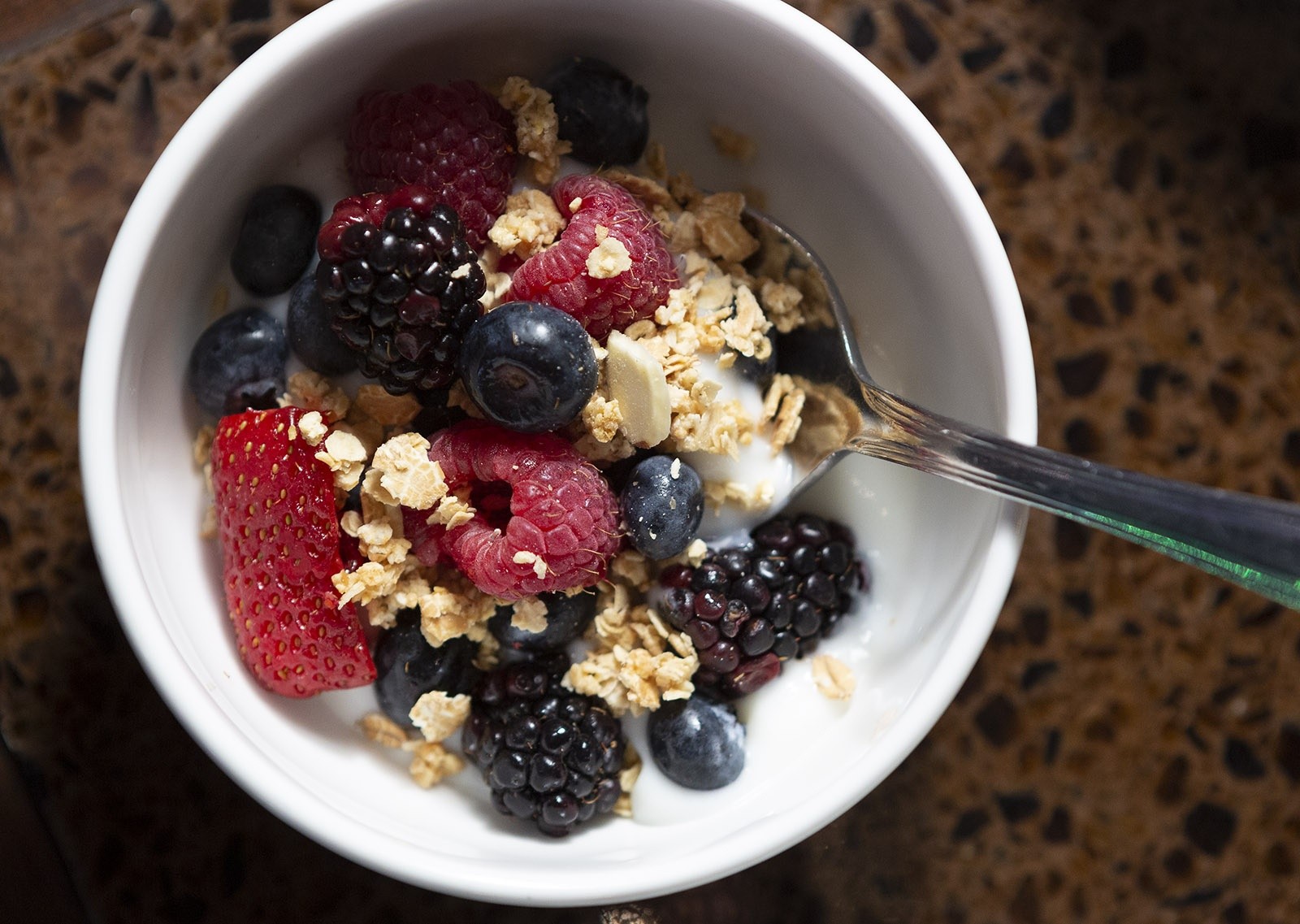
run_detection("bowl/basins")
[76,0,1040,913]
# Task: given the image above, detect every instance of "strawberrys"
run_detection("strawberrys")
[215,403,377,700]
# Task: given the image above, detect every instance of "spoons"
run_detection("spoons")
[694,190,1300,619]
